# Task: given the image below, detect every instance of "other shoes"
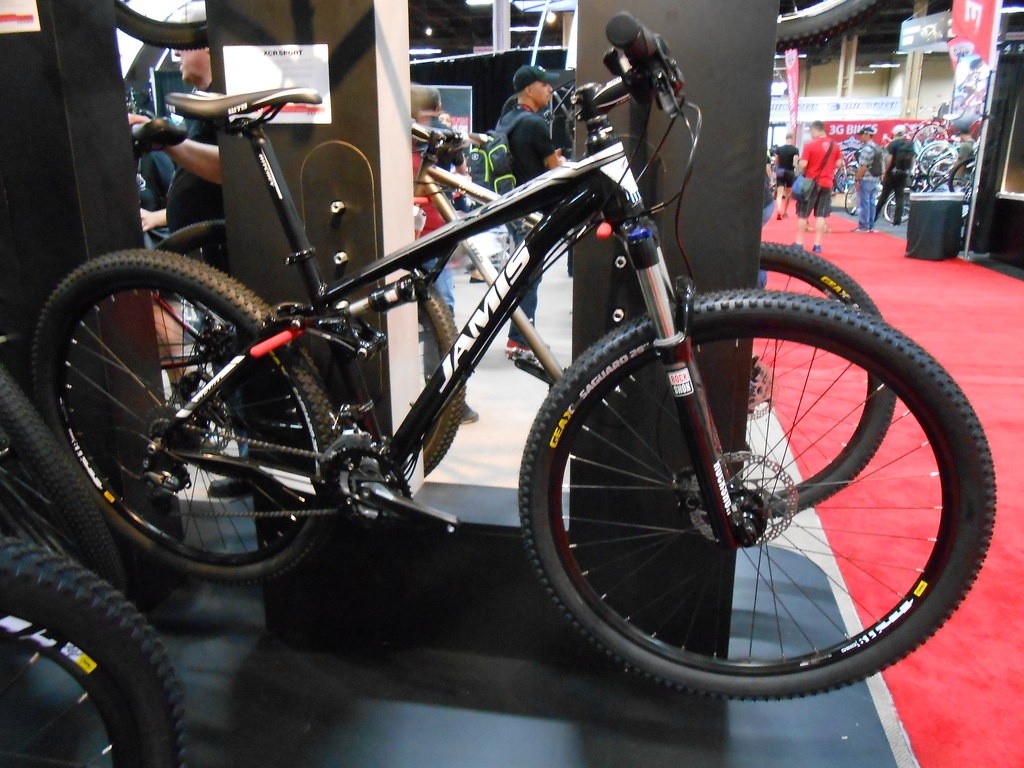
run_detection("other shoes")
[462,404,479,424]
[870,229,873,232]
[855,229,869,232]
[777,212,781,220]
[812,246,820,252]
[208,477,251,497]
[470,277,485,282]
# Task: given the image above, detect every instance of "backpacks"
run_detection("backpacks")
[866,144,883,176]
[466,111,531,195]
[896,142,914,169]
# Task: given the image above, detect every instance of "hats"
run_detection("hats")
[894,124,906,135]
[166,1,206,23]
[513,65,561,92]
[856,127,874,134]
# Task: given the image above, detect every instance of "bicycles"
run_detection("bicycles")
[0,8,999,768]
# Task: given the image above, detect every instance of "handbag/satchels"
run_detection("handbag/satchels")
[776,168,785,177]
[791,175,815,201]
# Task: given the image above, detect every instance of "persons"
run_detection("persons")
[128,2,253,497]
[411,63,568,424]
[756,113,982,292]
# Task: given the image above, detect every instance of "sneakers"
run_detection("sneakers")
[506,338,550,354]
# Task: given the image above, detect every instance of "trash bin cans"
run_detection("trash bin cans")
[906,192,965,261]
